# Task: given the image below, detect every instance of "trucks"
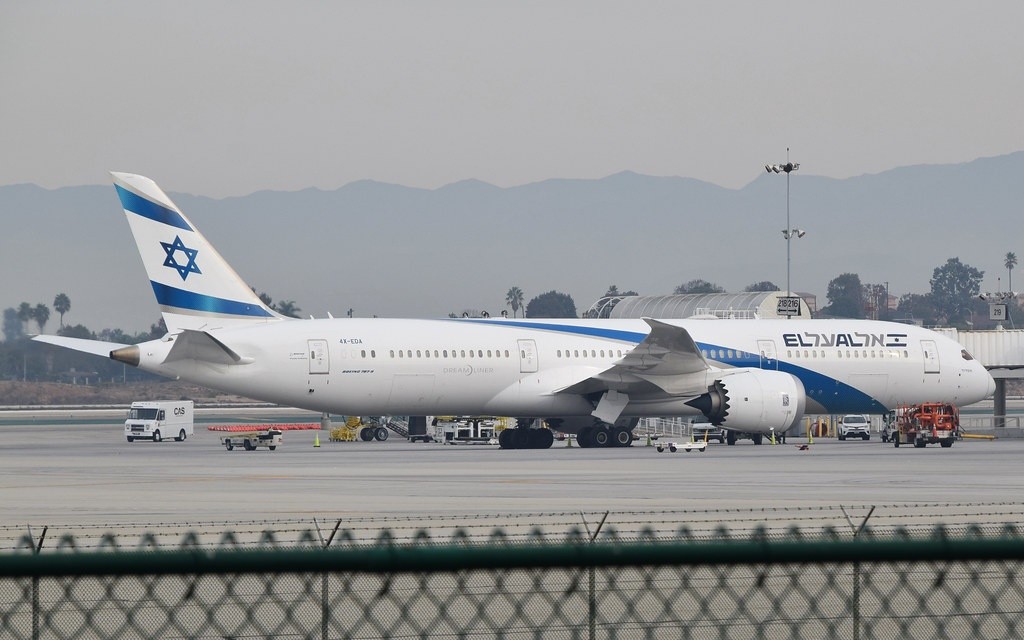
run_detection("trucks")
[124,400,194,442]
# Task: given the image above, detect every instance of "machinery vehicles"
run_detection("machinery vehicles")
[887,403,958,448]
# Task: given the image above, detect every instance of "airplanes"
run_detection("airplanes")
[30,171,998,451]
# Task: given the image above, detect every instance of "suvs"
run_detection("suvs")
[838,413,871,441]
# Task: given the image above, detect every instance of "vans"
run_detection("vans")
[690,412,728,444]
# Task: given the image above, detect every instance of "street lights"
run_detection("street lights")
[766,147,805,319]
[979,277,1017,330]
